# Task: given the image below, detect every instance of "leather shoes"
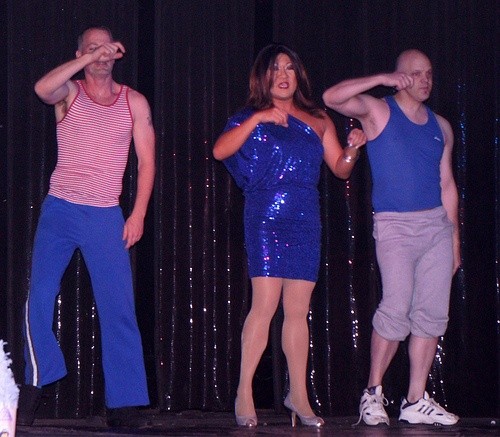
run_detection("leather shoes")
[18,385,41,425]
[106,408,153,429]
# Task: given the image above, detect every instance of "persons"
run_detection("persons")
[212,43,367,426]
[322,49,462,426]
[13,26,159,429]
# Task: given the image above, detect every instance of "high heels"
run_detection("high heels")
[235,398,257,427]
[284,392,325,427]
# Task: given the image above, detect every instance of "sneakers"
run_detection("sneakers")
[397,391,460,426]
[351,384,390,427]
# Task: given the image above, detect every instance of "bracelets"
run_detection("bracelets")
[342,146,360,162]
